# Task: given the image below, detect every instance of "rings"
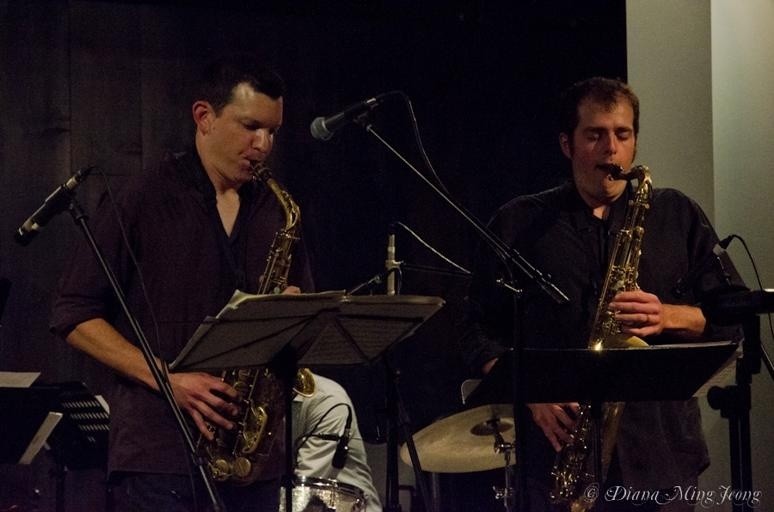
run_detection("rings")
[642,312,650,322]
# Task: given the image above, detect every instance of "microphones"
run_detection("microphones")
[310,88,401,141]
[669,235,734,300]
[13,166,89,246]
[331,410,353,469]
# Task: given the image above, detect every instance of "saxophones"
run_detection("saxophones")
[550,163,652,510]
[197,160,302,479]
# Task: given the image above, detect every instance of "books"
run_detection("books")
[166,284,445,366]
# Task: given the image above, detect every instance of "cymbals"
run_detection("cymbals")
[400,404,516,472]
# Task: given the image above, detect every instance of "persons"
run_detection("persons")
[45,56,319,511]
[452,74,753,512]
[276,369,381,511]
